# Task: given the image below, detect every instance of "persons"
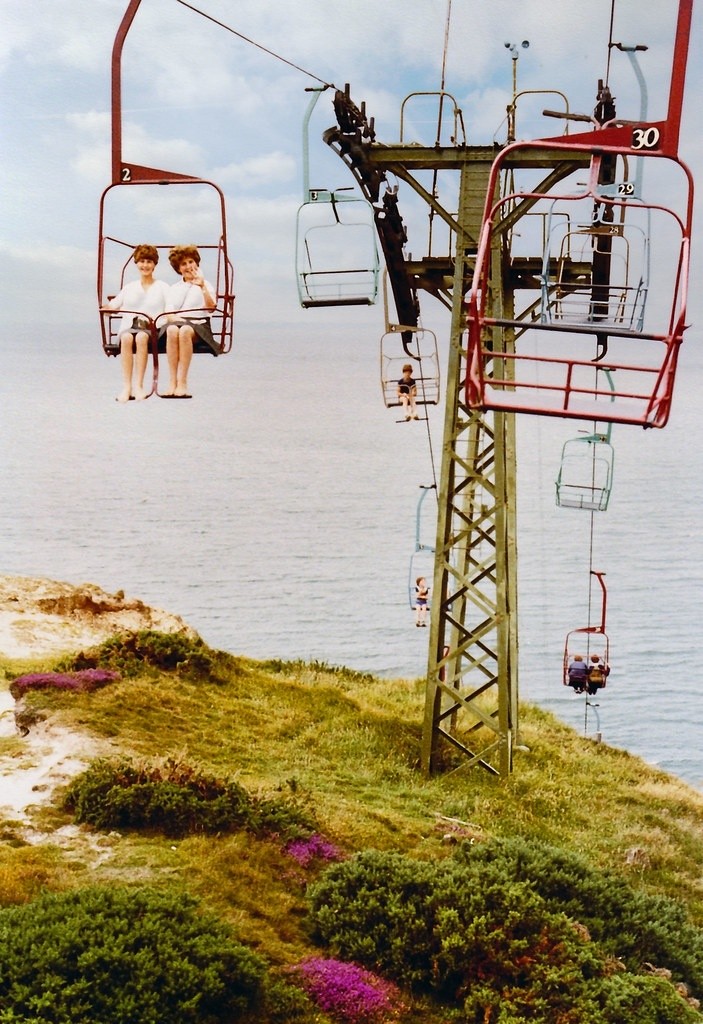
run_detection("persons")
[568,655,587,694]
[586,655,605,695]
[163,245,217,396]
[397,364,418,421]
[414,577,429,627]
[102,245,168,403]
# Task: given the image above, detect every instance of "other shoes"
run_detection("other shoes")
[574,689,583,693]
[588,691,596,695]
[416,621,426,627]
